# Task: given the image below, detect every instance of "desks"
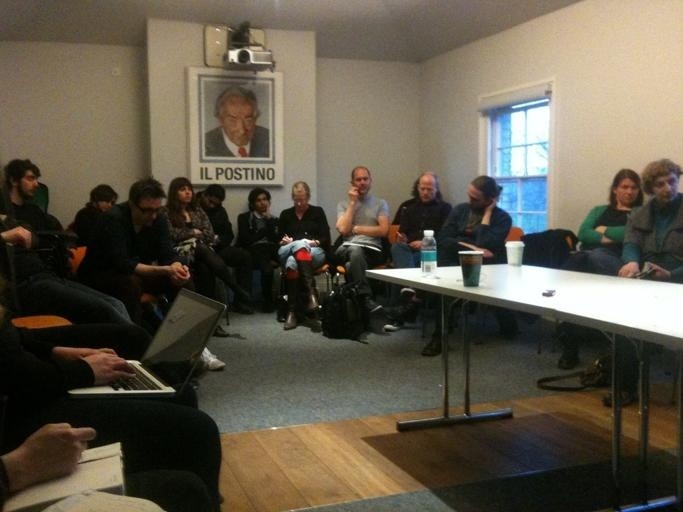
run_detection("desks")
[364,264,683,512]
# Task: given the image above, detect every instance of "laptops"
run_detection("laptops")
[67,287,227,400]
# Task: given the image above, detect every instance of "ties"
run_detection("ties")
[238,146,248,157]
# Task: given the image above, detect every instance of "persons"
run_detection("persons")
[204,86,270,158]
[279,166,512,357]
[0,159,284,511]
[550,159,682,408]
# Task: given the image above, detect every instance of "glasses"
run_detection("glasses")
[132,200,170,216]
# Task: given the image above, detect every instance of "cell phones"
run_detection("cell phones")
[350,180,358,187]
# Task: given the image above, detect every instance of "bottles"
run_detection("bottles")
[420,229,438,277]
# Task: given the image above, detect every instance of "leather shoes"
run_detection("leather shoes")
[304,293,323,315]
[282,311,298,330]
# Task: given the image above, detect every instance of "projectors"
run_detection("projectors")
[226,48,275,70]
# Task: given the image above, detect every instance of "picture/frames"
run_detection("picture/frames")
[183,66,285,188]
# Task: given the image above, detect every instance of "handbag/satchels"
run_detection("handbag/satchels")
[321,278,371,340]
[581,352,621,389]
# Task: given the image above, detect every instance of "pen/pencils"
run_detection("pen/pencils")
[397,232,403,238]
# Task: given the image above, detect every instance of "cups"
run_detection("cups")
[505,240,525,267]
[457,250,485,287]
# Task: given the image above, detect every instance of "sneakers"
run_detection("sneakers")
[199,344,226,371]
[361,295,384,315]
[601,386,639,407]
[556,346,580,371]
[384,319,406,332]
[399,286,419,297]
[232,286,252,301]
[421,336,447,356]
[229,301,255,315]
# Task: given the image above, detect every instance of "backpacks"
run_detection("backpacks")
[519,228,578,270]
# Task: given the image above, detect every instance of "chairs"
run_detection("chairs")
[334,224,400,320]
[521,230,576,353]
[23,182,49,214]
[138,291,159,305]
[280,263,330,321]
[422,226,524,350]
[37,230,88,273]
[0,241,74,332]
